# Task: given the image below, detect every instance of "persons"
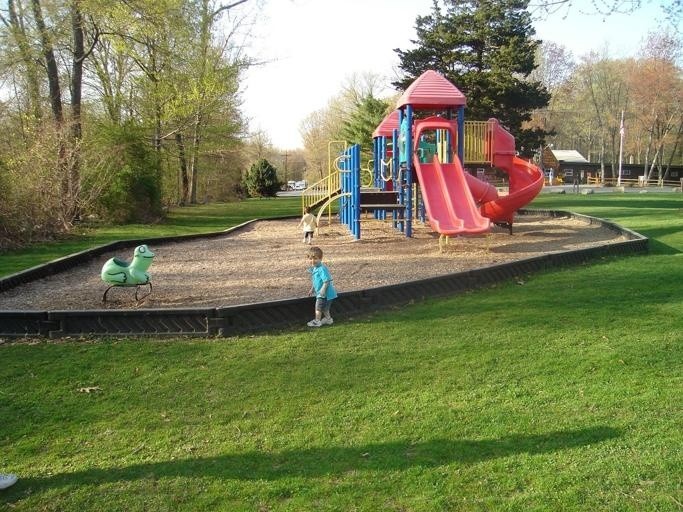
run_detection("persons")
[304,246,337,328]
[296,207,318,245]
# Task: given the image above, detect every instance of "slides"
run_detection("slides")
[478,119,544,225]
[414,153,490,235]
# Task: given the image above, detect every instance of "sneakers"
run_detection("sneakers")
[306,320,322,327]
[321,317,333,325]
